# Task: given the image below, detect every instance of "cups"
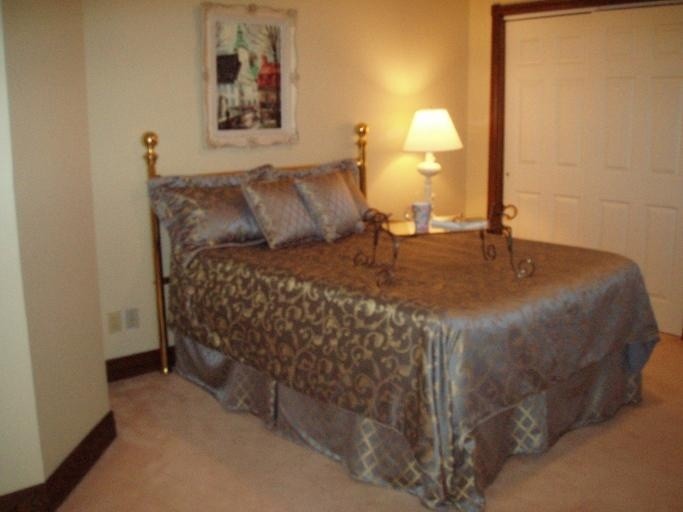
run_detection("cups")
[409,202,432,236]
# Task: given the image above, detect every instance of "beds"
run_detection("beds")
[143,124,661,512]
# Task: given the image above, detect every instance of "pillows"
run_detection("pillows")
[147,159,371,266]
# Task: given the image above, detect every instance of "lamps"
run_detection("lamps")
[405,108,464,203]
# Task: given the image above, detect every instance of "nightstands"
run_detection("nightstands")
[378,215,489,239]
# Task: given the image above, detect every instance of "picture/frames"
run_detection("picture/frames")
[203,3,297,146]
